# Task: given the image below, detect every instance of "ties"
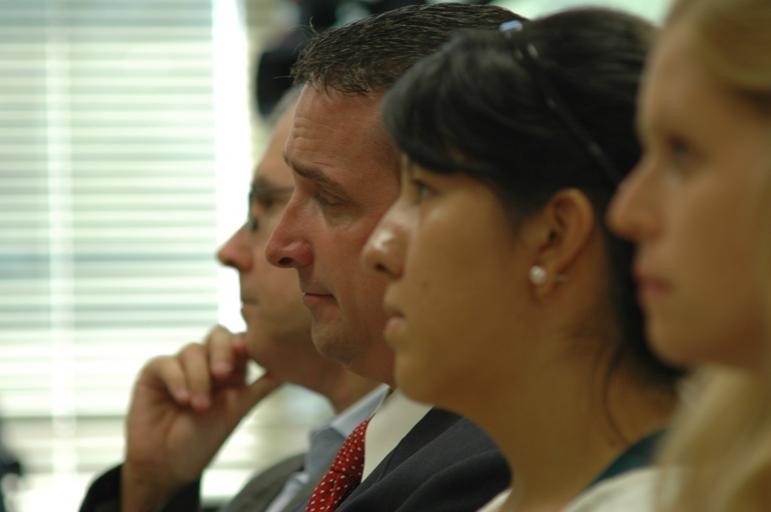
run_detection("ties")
[304,413,376,512]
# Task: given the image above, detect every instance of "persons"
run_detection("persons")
[605,1,770,512]
[284,1,532,512]
[77,82,391,512]
[362,6,682,512]
